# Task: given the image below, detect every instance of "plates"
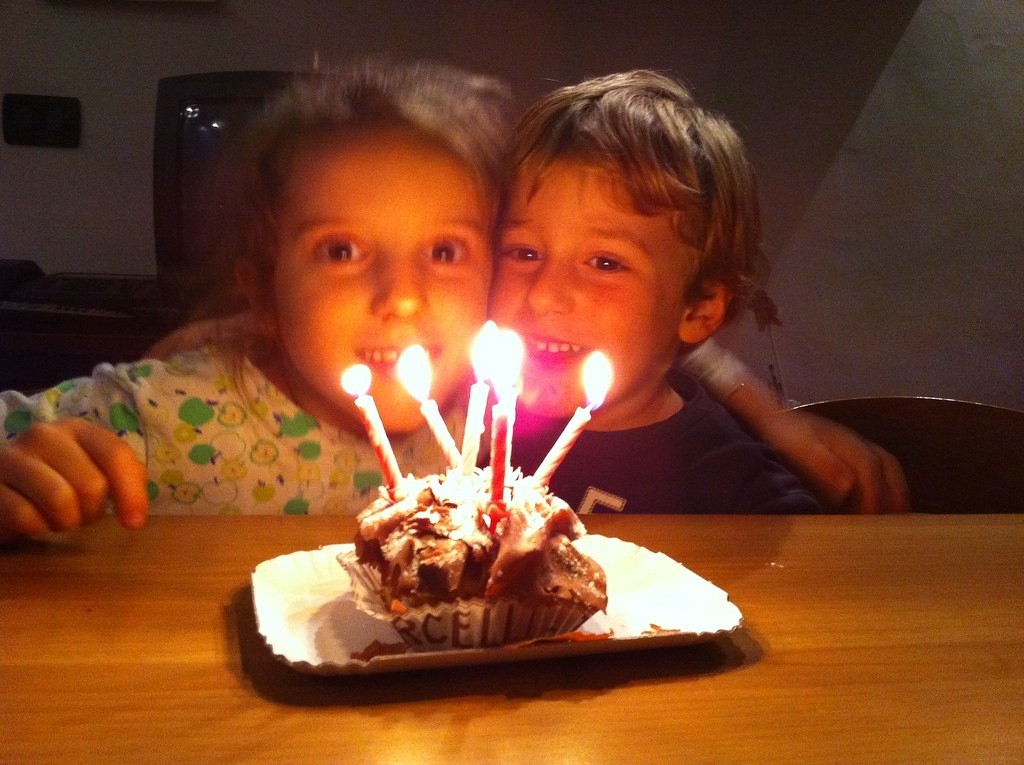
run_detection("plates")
[251,533,744,675]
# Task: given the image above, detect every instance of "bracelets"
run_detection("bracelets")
[681,339,744,400]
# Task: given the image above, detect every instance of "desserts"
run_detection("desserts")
[336,461,608,651]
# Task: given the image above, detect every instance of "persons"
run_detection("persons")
[0,62,908,537]
[144,70,824,514]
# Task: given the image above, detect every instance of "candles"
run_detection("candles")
[491,400,515,500]
[356,393,407,500]
[421,400,469,479]
[533,404,593,486]
[458,384,490,475]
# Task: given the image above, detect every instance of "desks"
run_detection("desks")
[1,508,1024,765]
